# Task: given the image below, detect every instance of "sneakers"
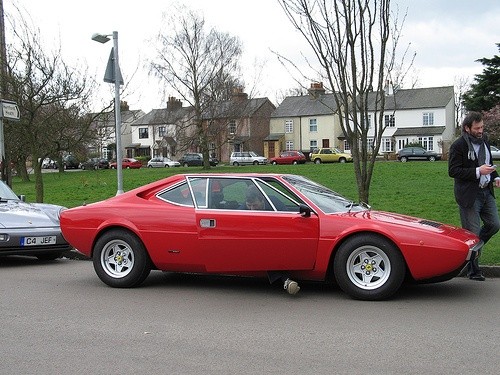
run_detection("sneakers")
[283,278,301,296]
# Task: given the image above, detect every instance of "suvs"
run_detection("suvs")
[308,147,355,164]
[180,152,219,168]
[230,150,269,165]
[396,145,442,164]
[489,145,500,161]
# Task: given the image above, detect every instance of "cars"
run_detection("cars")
[79,157,108,169]
[270,150,306,166]
[33,158,54,169]
[108,157,142,171]
[52,154,79,171]
[147,157,181,168]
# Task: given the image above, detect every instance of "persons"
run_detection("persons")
[238,185,301,296]
[449,112,500,281]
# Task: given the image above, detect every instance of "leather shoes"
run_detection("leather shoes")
[469,274,487,281]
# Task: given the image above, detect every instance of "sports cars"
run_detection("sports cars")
[59,171,485,301]
[0,177,71,263]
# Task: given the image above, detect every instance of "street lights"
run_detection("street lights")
[91,28,126,195]
